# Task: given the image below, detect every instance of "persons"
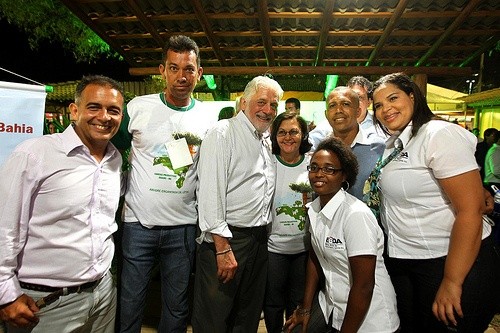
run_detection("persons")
[368,72,500,333]
[308,76,392,152]
[286,98,316,132]
[325,86,495,258]
[262,111,318,333]
[471,127,500,213]
[283,137,400,333]
[110,35,218,333]
[191,76,285,333]
[0,75,128,333]
[46,112,77,134]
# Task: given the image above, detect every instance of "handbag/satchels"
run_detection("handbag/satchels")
[482,215,495,240]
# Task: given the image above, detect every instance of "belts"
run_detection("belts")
[150,225,195,230]
[18,277,103,309]
[228,224,268,232]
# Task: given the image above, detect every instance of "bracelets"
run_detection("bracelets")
[296,305,310,317]
[216,248,232,255]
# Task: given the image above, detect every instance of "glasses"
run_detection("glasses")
[307,164,343,175]
[277,128,302,136]
